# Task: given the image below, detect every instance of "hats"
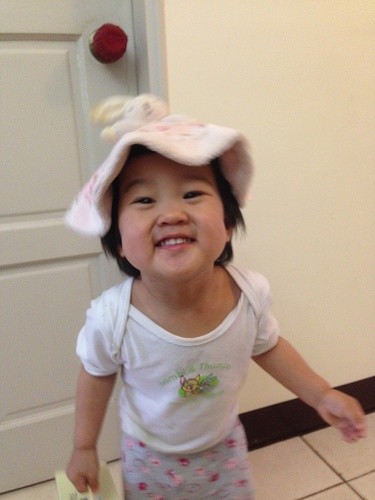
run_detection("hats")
[64,94,255,235]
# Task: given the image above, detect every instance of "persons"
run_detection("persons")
[64,93,368,499]
